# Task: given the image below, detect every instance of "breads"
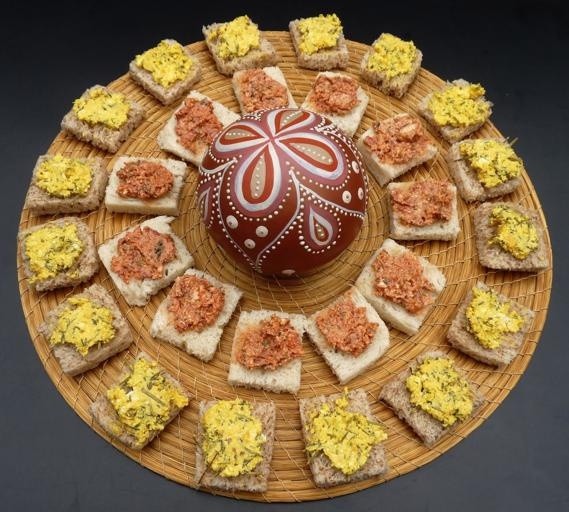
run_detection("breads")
[18,12,549,494]
